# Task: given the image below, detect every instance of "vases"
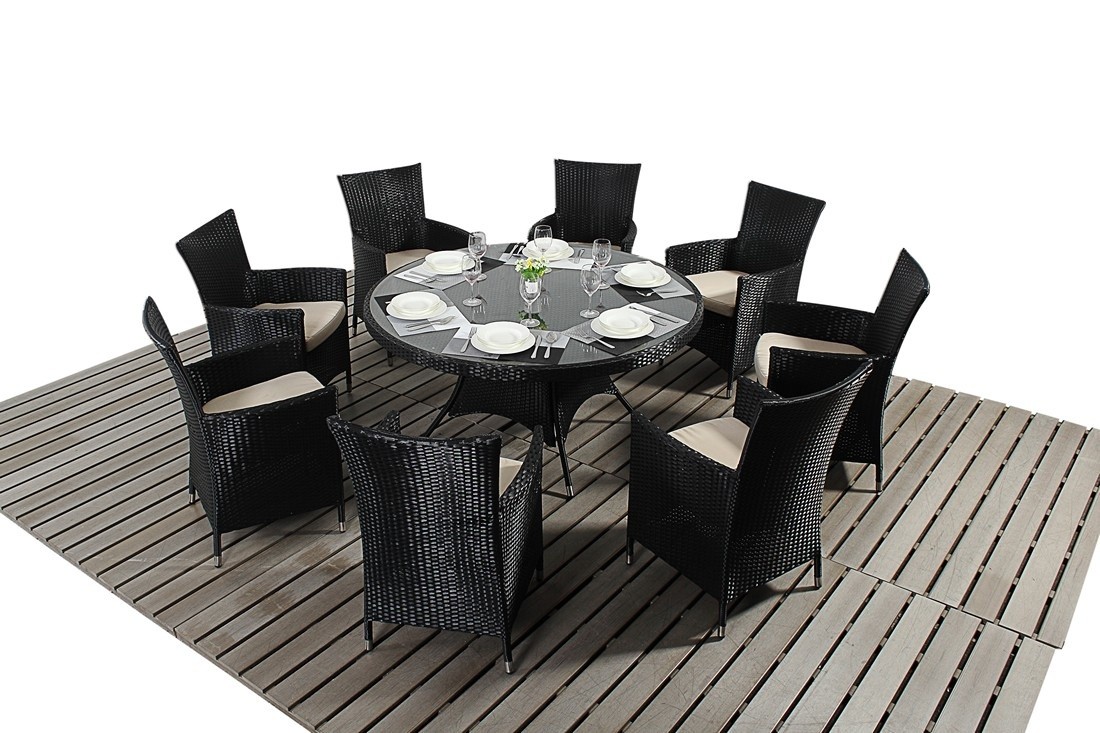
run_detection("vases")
[524,297,540,313]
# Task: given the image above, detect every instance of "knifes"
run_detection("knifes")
[506,245,518,259]
[629,306,680,324]
[531,335,541,359]
[574,248,585,264]
[410,270,446,282]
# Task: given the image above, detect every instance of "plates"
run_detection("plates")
[522,248,574,261]
[470,330,536,354]
[614,270,672,288]
[590,317,655,339]
[422,257,476,275]
[385,300,448,320]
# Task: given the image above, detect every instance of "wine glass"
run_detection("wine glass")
[463,225,612,328]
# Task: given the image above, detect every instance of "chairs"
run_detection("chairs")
[666,182,825,398]
[143,296,347,568]
[176,209,352,394]
[528,159,641,255]
[627,362,872,640]
[337,163,476,366]
[326,410,546,673]
[754,251,930,491]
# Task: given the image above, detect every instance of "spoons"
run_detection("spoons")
[543,331,557,358]
[408,316,455,332]
[513,245,525,257]
[403,274,436,283]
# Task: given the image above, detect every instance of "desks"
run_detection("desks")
[364,242,704,498]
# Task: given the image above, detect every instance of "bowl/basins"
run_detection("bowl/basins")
[598,307,650,334]
[424,250,469,272]
[390,291,440,317]
[478,321,531,351]
[620,263,666,284]
[526,238,568,257]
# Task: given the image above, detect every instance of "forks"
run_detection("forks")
[636,290,677,297]
[576,328,616,349]
[460,326,477,353]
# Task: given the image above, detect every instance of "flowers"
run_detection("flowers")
[517,310,548,330]
[515,255,549,282]
